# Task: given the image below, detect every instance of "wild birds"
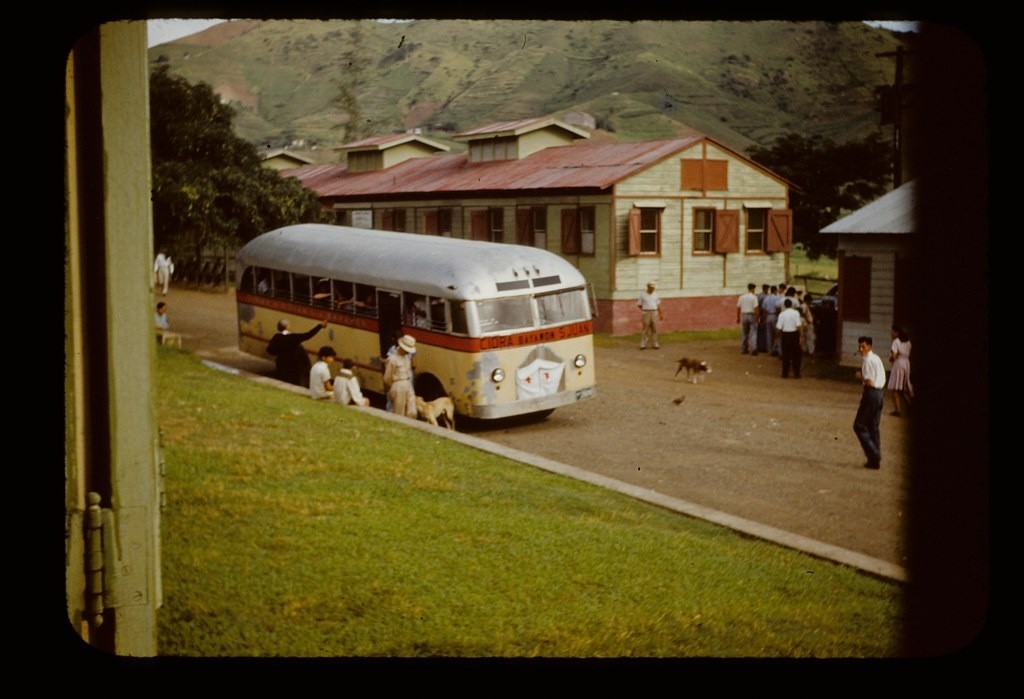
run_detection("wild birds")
[397,35,406,49]
[521,35,527,49]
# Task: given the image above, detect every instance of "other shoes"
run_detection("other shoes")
[653,344,659,348]
[781,374,788,378]
[890,411,904,417]
[741,350,748,354]
[751,351,758,355]
[869,454,882,464]
[640,344,646,349]
[794,374,801,378]
[864,461,881,470]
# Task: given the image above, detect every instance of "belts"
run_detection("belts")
[642,309,658,311]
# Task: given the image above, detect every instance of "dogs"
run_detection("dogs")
[416,396,455,431]
[675,357,713,384]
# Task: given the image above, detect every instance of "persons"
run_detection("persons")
[887,326,912,417]
[379,330,416,412]
[155,302,170,331]
[637,282,664,350]
[331,359,370,407]
[154,244,175,296]
[891,323,914,398]
[855,336,886,469]
[267,317,328,388]
[736,283,840,379]
[383,334,418,420]
[309,344,337,400]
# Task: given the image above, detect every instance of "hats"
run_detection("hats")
[647,282,654,288]
[398,334,417,353]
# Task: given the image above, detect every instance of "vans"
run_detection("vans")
[235,221,599,433]
